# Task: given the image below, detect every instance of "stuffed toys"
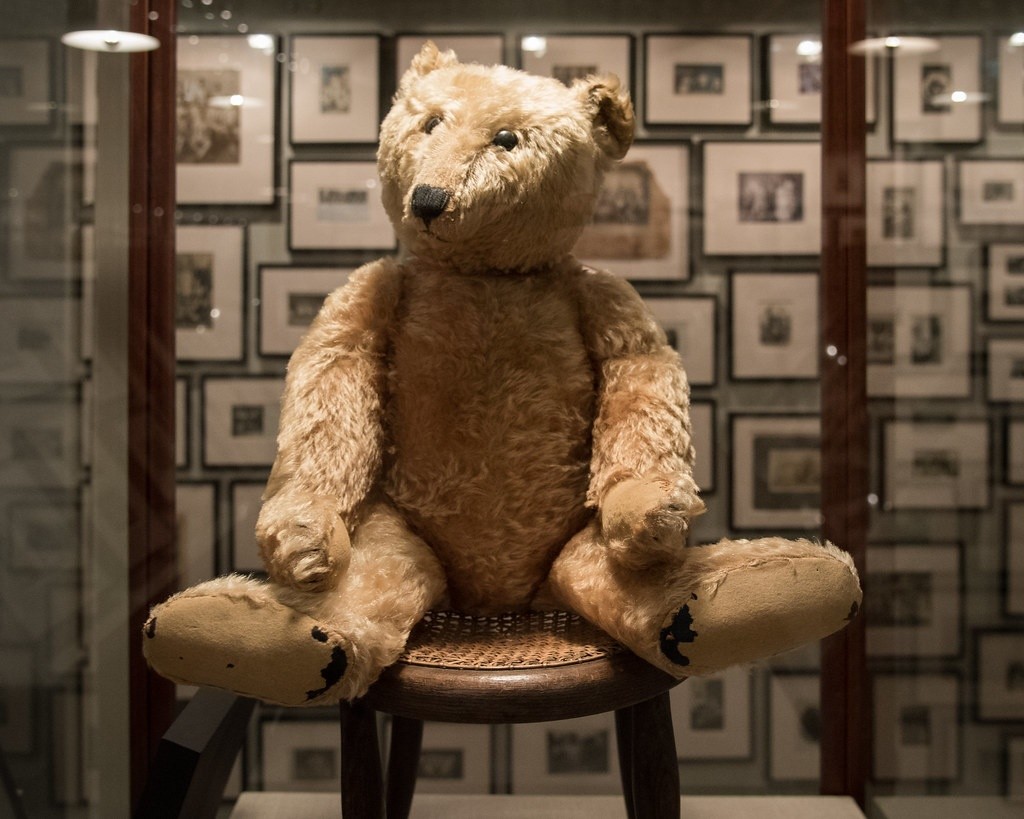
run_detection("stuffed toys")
[139,47,865,706]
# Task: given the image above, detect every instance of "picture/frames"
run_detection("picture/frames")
[0,27,1024,807]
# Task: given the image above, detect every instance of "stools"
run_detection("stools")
[337,610,689,819]
